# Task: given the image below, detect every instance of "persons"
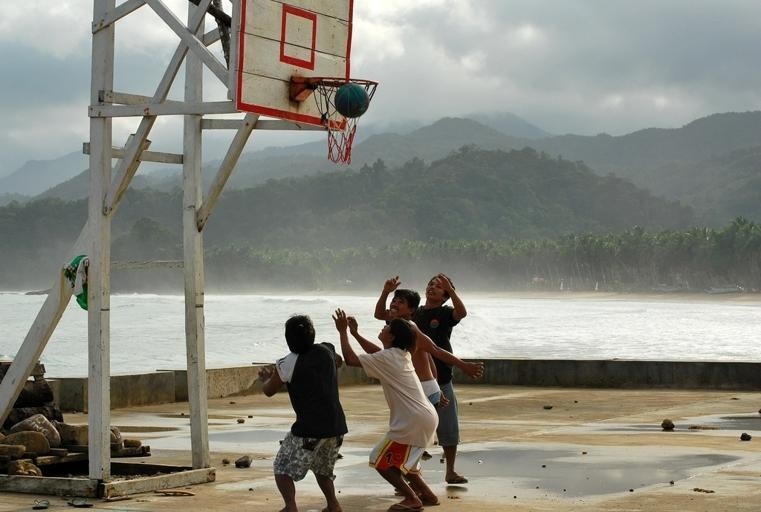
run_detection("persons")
[389,288,485,497]
[259,314,350,512]
[375,272,470,485]
[332,306,442,512]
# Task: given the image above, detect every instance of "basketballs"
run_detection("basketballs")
[335,83,370,118]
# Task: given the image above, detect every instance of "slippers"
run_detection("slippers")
[447,475,468,483]
[417,494,440,506]
[388,503,425,512]
